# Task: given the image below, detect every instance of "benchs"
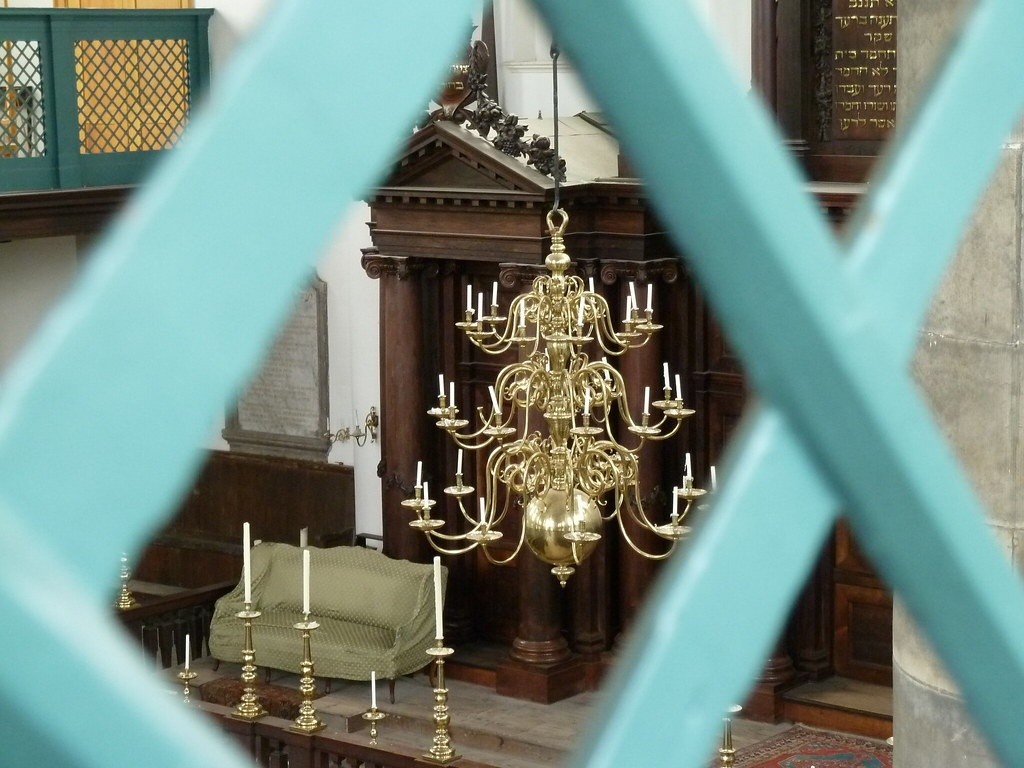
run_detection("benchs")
[208,541,450,704]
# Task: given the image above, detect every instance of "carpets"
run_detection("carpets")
[709,725,893,768]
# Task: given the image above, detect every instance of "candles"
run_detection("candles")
[243,521,252,603]
[433,556,443,638]
[302,548,310,612]
[372,671,377,708]
[185,634,190,669]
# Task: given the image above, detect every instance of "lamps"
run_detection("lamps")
[399,32,718,589]
[323,406,378,447]
